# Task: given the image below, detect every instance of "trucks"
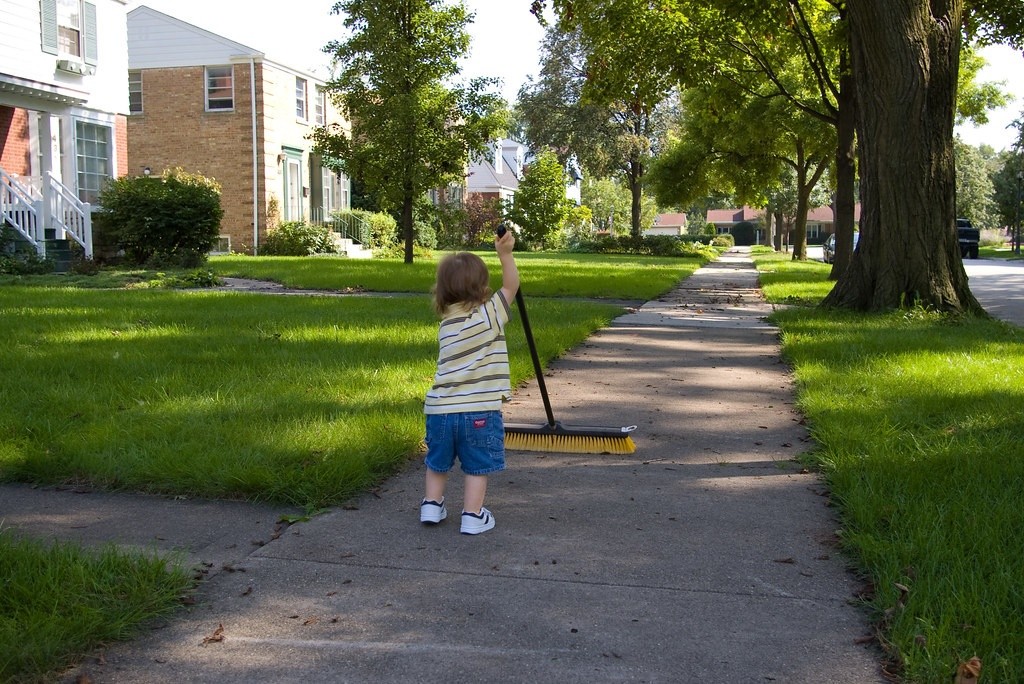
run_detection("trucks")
[956,218,980,259]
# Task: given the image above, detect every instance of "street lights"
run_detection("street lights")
[610,205,614,238]
[1016,170,1023,253]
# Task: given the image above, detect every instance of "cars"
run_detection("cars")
[824,232,860,262]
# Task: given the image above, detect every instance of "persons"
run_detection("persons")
[420,232,519,534]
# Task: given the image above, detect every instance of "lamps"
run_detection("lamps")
[278,152,287,164]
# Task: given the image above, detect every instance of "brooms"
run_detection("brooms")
[497,225,638,454]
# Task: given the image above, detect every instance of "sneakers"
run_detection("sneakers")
[460,507,495,535]
[421,496,447,524]
